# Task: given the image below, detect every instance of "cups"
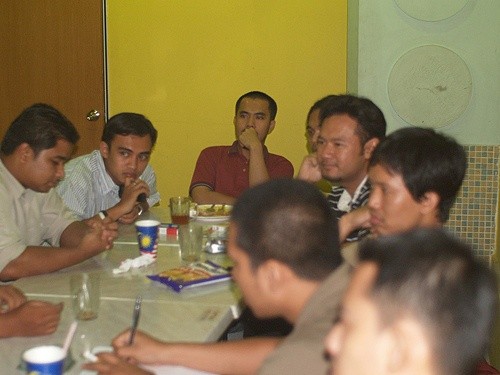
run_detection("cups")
[70,268,100,320]
[21,345,68,375]
[135,219,160,261]
[178,224,203,261]
[168,196,190,225]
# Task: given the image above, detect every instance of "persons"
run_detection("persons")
[189,91,295,205]
[53,112,161,225]
[322,225,500,375]
[298,95,387,243]
[338,126,469,263]
[0,284,64,339]
[306,96,337,153]
[0,101,120,282]
[82,177,354,375]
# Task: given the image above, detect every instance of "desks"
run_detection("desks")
[0,208,245,375]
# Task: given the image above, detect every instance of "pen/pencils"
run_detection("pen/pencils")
[161,223,180,228]
[126,296,142,361]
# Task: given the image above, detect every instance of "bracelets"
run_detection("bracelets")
[98,210,107,220]
[136,203,145,216]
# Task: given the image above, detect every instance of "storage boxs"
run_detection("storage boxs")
[158,222,179,235]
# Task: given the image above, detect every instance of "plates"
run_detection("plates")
[194,204,233,222]
